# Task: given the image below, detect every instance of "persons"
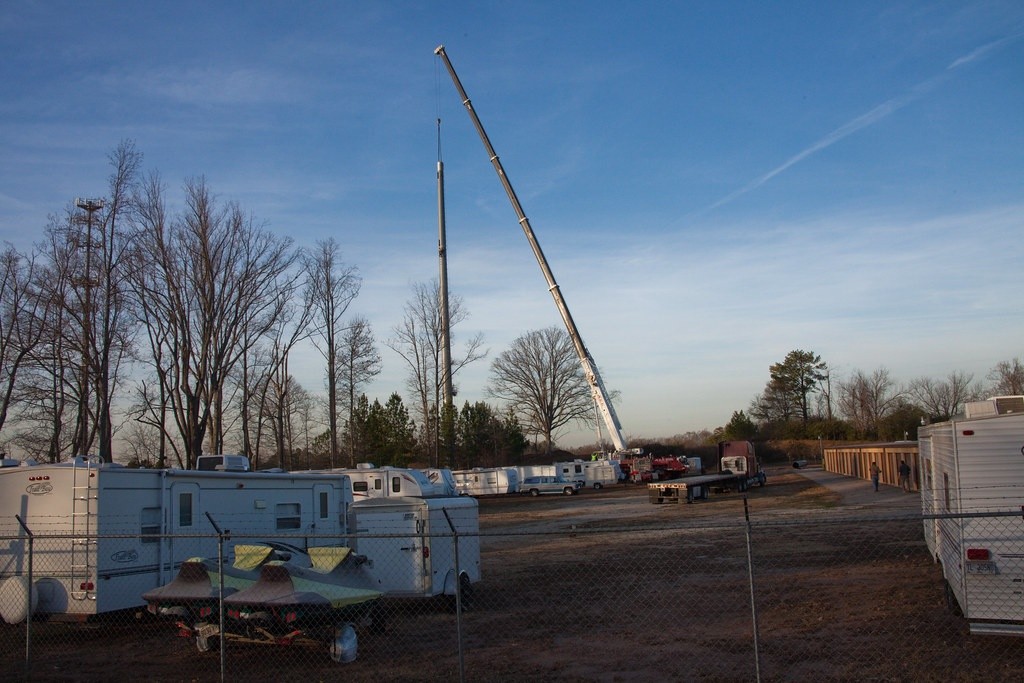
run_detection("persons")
[898,460,911,492]
[869,462,881,493]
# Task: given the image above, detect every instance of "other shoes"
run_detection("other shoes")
[903,491,911,494]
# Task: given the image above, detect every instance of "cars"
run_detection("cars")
[520,476,584,497]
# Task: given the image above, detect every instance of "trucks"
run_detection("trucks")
[0,455,627,635]
[918,394,1024,638]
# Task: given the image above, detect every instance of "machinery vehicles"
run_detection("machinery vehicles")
[435,44,690,481]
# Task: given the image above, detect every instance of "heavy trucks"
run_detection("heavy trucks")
[646,441,768,505]
